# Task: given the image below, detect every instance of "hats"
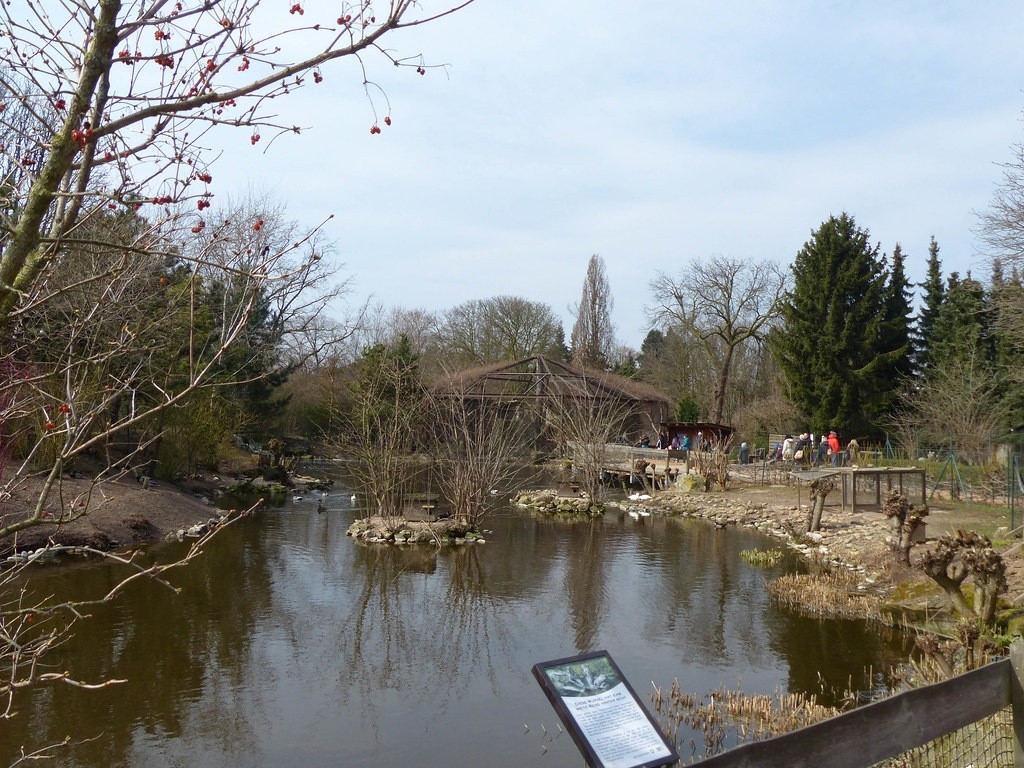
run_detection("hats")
[741,442,748,448]
[698,432,703,436]
[799,434,804,441]
[774,442,779,449]
[848,439,860,448]
[830,431,836,435]
[821,436,827,443]
[804,432,808,439]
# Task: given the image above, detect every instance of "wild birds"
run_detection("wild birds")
[292,490,358,514]
[490,489,500,495]
[627,492,654,502]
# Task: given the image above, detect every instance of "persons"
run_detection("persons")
[656,431,690,450]
[640,434,650,448]
[763,431,839,467]
[738,443,749,465]
[842,439,861,467]
